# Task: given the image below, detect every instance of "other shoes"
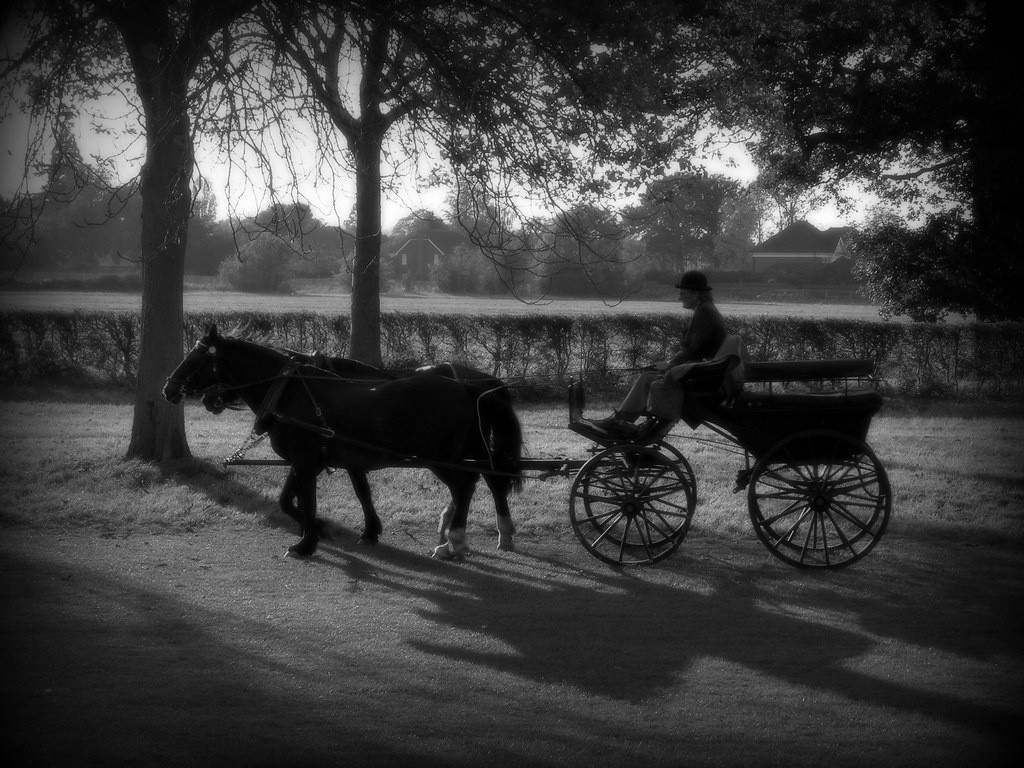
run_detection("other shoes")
[589,407,635,435]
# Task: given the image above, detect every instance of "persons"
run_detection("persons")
[591,270,728,439]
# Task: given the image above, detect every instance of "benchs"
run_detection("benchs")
[735,359,882,412]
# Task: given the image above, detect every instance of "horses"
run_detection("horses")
[163,323,523,562]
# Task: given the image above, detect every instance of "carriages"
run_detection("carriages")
[163,321,892,572]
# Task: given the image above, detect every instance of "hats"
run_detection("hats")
[675,271,713,292]
